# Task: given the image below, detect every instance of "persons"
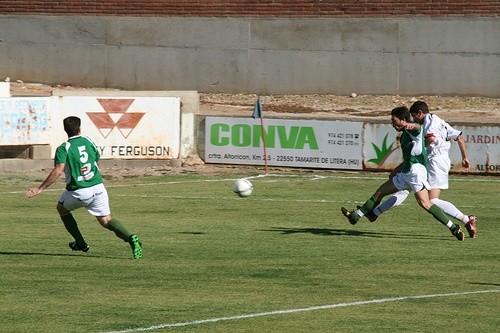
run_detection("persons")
[355,101,477,238]
[341,107,466,242]
[25,116,144,259]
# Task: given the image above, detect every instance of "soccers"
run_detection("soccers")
[232,178,256,198]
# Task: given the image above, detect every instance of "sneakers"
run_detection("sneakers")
[129,234,142,260]
[69,242,89,252]
[341,207,357,225]
[465,215,476,238]
[451,224,464,241]
[357,205,378,222]
